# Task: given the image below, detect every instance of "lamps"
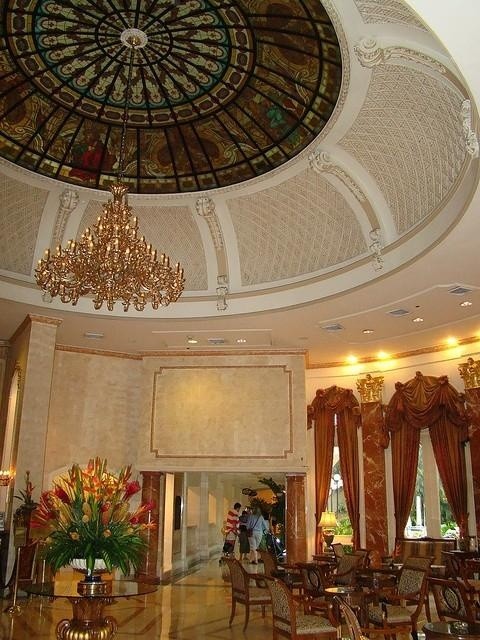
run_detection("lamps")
[34,36,184,312]
[317,512,339,555]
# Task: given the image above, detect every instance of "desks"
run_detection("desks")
[14,580,157,640]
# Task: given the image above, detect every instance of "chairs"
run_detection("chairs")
[222,538,479,640]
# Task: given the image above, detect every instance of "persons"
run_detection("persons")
[222,503,270,564]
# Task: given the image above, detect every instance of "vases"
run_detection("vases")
[70,558,113,574]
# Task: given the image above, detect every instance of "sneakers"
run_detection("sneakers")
[250,558,264,565]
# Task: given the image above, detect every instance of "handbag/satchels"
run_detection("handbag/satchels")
[247,528,254,537]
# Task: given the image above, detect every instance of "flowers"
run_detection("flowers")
[24,457,157,577]
